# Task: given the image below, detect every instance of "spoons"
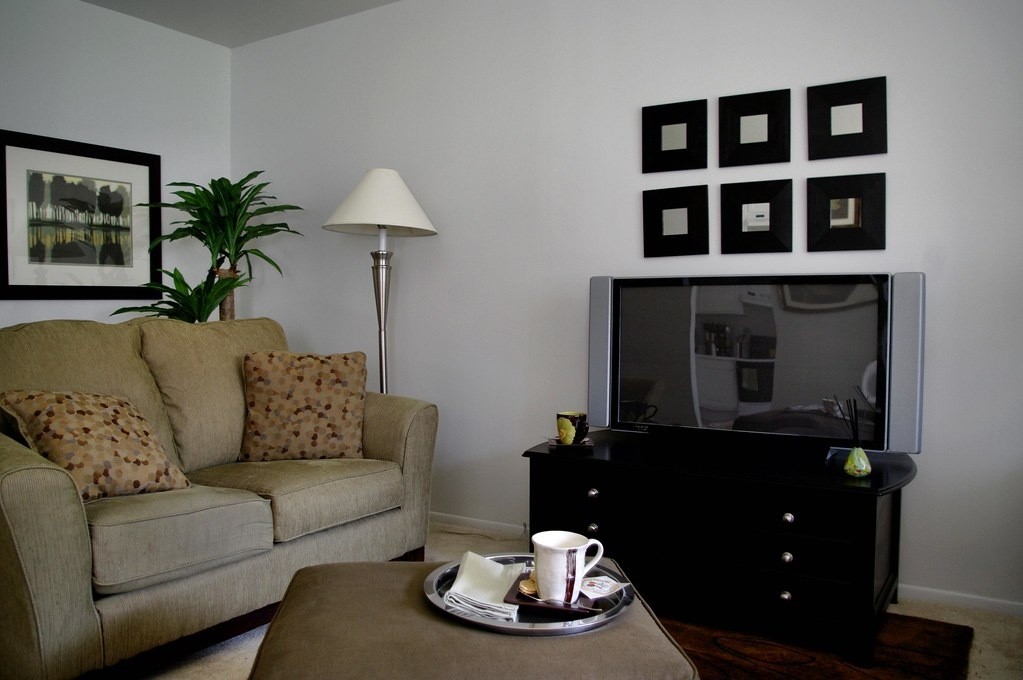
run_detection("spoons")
[519,590,602,611]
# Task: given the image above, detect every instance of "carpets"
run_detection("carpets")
[631,574,976,680]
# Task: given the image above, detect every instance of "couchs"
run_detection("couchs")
[0,317,439,680]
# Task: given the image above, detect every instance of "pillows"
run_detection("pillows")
[0,389,192,505]
[239,349,367,458]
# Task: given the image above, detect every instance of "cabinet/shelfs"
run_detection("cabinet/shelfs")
[522,431,918,664]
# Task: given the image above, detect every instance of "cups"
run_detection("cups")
[823,398,842,415]
[620,400,657,423]
[557,412,589,445]
[532,530,604,604]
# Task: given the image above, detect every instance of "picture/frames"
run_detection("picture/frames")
[642,78,888,255]
[0,129,162,302]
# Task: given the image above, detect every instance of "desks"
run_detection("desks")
[249,558,699,680]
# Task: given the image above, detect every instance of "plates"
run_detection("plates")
[547,439,594,447]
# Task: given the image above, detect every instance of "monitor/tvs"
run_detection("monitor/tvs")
[585,272,925,454]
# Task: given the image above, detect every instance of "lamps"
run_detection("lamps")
[321,170,439,396]
